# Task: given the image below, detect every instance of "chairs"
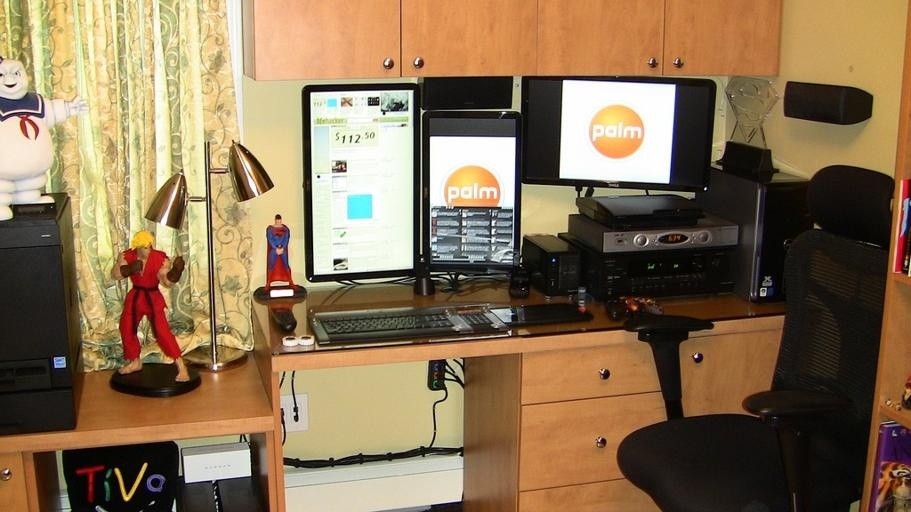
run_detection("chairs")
[615,165,894,510]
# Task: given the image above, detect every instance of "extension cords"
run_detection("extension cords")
[427,360,446,391]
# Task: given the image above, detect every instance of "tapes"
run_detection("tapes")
[282,336,298,346]
[299,335,315,346]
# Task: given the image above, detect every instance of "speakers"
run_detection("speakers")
[0,194,85,437]
[784,81,874,125]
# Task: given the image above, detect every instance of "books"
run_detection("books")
[893,177,911,278]
[868,422,911,512]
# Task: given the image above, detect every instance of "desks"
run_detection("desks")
[2,354,278,511]
[251,266,798,512]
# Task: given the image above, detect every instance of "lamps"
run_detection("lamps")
[144,136,276,370]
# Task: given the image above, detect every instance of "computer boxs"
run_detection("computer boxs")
[697,156,811,304]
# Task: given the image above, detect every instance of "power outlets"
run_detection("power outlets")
[271,398,308,433]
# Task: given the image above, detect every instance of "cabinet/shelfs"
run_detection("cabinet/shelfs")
[862,1,910,510]
[536,0,781,80]
[243,3,536,79]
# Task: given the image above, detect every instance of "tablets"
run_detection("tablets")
[491,303,594,327]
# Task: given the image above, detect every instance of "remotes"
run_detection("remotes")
[270,305,297,332]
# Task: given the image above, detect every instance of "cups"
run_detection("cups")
[508,273,532,299]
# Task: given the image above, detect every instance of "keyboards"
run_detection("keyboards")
[309,302,509,346]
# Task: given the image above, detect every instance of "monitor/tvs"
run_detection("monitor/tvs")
[423,110,521,272]
[520,76,718,193]
[301,82,419,283]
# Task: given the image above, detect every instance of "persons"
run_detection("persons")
[265,214,299,294]
[109,231,190,382]
